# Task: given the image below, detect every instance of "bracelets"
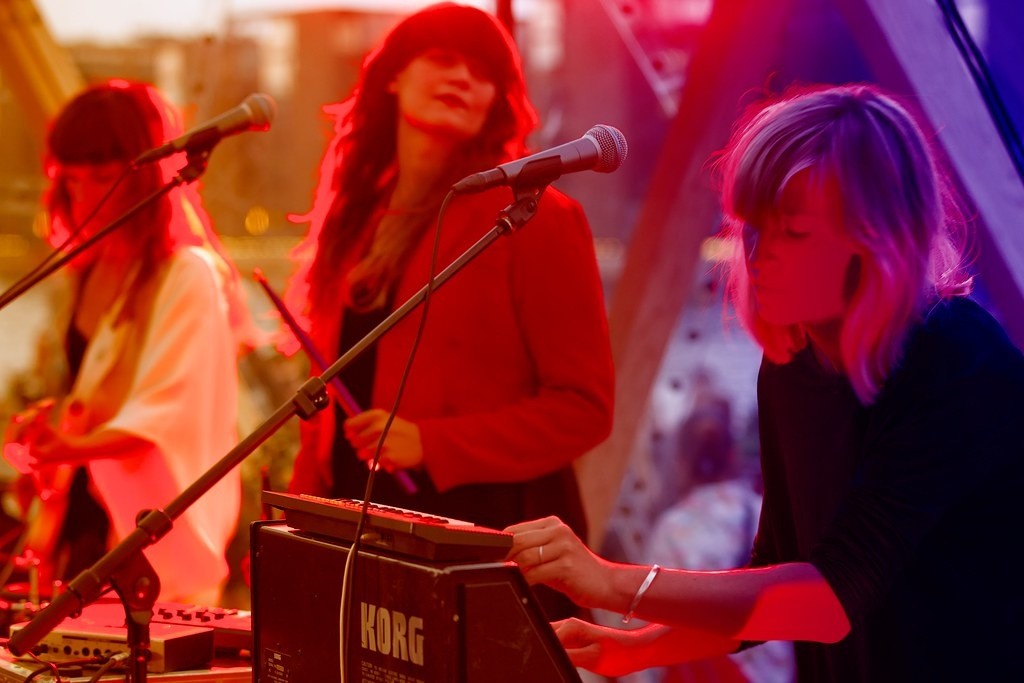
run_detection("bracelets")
[621,563,660,623]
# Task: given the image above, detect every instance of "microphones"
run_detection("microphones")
[452,125,628,197]
[132,94,277,166]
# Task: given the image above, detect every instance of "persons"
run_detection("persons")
[10,80,243,612]
[489,82,1024,683]
[289,0,617,633]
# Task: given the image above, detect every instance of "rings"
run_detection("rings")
[539,545,544,564]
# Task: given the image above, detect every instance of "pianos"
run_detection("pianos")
[259,484,516,563]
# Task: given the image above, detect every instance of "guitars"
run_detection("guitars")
[22,393,90,559]
